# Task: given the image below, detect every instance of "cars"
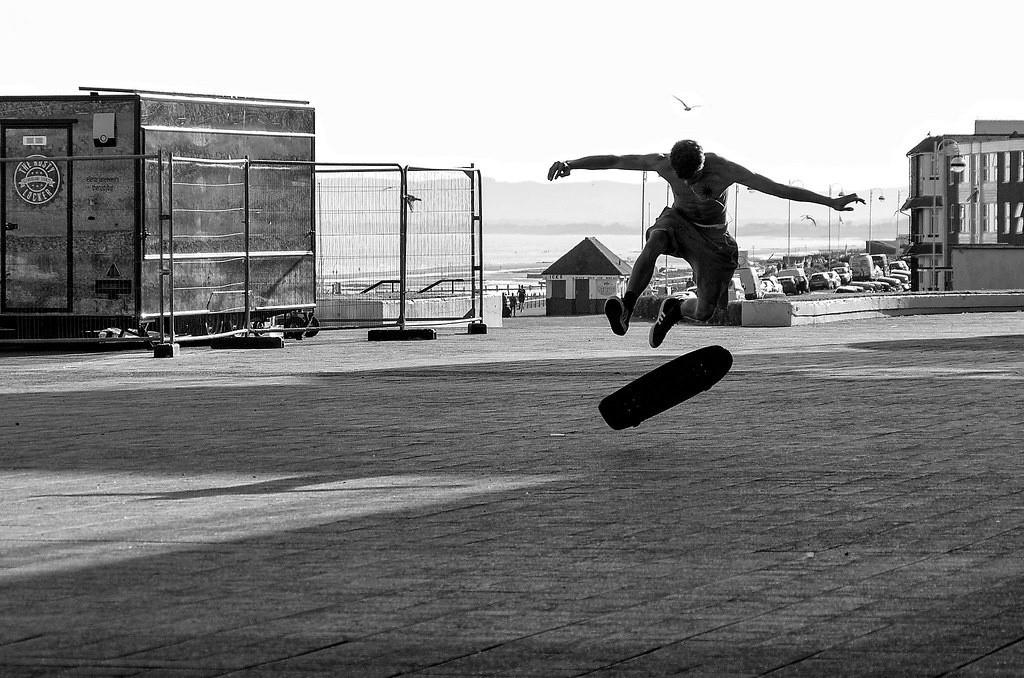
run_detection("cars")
[735,251,912,296]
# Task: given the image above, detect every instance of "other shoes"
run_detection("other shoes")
[650,298,683,348]
[605,295,630,336]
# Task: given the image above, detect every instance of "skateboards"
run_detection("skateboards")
[598,345,734,430]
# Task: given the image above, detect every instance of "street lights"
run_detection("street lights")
[734,182,757,243]
[827,182,846,271]
[868,188,888,254]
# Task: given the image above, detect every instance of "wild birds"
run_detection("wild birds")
[671,94,703,112]
[381,185,401,192]
[800,212,817,227]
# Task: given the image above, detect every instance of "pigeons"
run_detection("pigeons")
[927,130,931,137]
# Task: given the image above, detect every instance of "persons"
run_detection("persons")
[517,285,526,312]
[502,293,508,308]
[546,140,866,348]
[509,292,518,317]
